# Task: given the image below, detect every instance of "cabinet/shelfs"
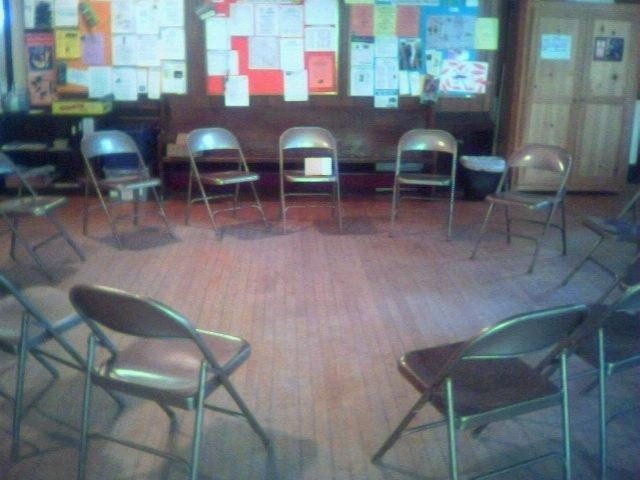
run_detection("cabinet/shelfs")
[4,100,165,195]
[500,0,640,195]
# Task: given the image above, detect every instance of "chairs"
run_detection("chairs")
[276,126,345,233]
[553,188,640,304]
[366,304,589,480]
[75,125,175,252]
[69,282,271,480]
[0,265,127,461]
[0,150,86,279]
[182,123,271,240]
[467,144,571,272]
[535,272,639,480]
[389,127,463,239]
[154,88,440,199]
